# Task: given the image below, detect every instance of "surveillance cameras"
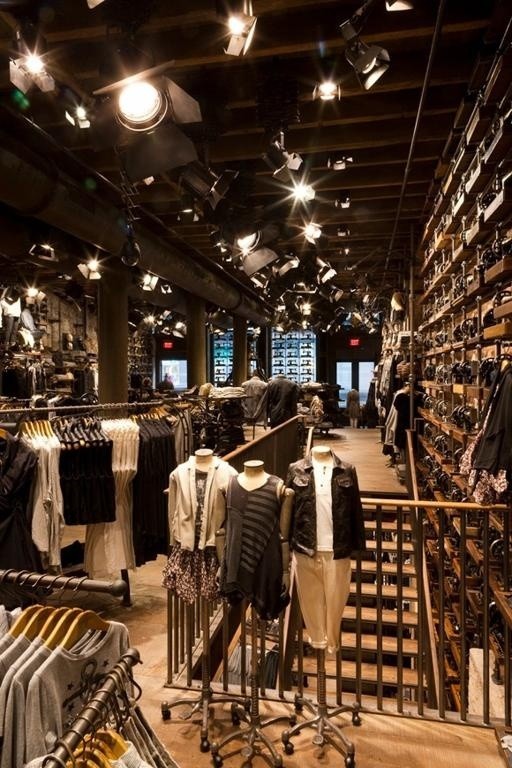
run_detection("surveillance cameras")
[120,239,141,267]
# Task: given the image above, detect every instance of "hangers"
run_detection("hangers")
[1,401,183,451]
[0,570,131,684]
[32,656,147,768]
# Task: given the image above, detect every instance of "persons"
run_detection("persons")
[156,374,174,395]
[220,458,294,619]
[167,447,238,604]
[288,446,365,656]
[239,373,268,426]
[268,373,299,431]
[346,388,362,429]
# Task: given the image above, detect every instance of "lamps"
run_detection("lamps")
[78,259,104,280]
[140,273,157,295]
[341,20,392,88]
[225,2,259,56]
[314,78,344,102]
[92,57,202,131]
[6,49,60,93]
